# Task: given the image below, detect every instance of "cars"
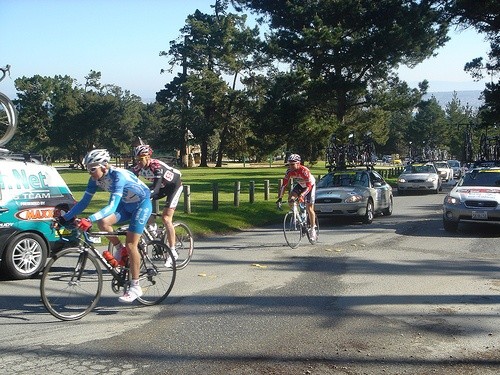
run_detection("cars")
[434,161,453,183]
[397,163,443,193]
[443,167,500,232]
[311,170,393,225]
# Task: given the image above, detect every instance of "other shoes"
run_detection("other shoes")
[118,285,143,304]
[114,248,123,262]
[310,228,316,238]
[164,252,178,268]
[146,223,158,231]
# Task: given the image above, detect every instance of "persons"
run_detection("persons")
[276,153,316,241]
[50,148,153,302]
[129,144,183,267]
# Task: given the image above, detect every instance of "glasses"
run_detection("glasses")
[87,165,102,172]
[290,163,297,165]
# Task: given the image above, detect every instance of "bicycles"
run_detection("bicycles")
[276,197,319,249]
[0,64,17,148]
[41,212,178,320]
[108,192,193,271]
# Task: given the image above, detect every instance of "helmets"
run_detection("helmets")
[133,145,153,157]
[81,148,111,169]
[288,154,301,161]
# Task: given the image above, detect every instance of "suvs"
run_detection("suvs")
[0,152,79,280]
[447,160,461,179]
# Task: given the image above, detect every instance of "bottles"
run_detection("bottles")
[120,247,128,267]
[147,225,157,238]
[102,251,119,267]
[156,226,164,239]
